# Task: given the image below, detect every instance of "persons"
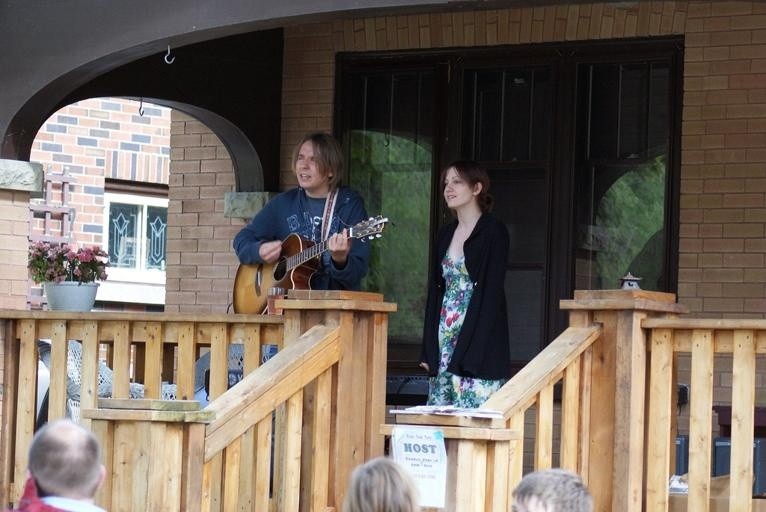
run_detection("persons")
[506,464,594,512]
[231,131,371,291]
[0,419,115,512]
[416,160,510,402]
[341,454,423,512]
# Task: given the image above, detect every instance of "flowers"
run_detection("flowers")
[28,241,110,286]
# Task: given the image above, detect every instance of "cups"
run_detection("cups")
[267,287,284,316]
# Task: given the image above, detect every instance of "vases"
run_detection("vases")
[42,281,100,311]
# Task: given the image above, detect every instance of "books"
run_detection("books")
[385,402,505,422]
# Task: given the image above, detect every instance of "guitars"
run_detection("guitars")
[233,215,389,316]
